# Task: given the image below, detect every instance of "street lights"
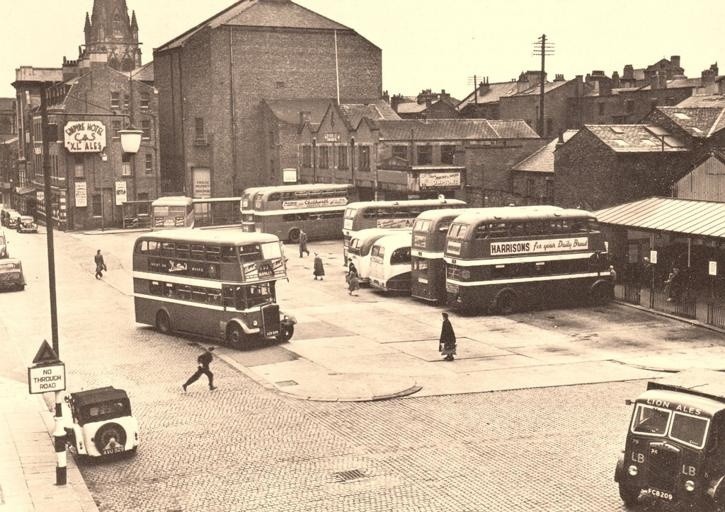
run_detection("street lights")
[37,99,145,456]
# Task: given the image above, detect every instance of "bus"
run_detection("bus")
[132,230,295,349]
[256,184,361,242]
[370,234,411,294]
[240,185,260,233]
[345,228,413,287]
[152,194,195,231]
[445,209,616,314]
[409,206,565,304]
[344,196,467,247]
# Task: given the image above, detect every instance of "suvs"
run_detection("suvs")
[62,388,140,460]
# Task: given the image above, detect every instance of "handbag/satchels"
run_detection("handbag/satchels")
[444,343,455,350]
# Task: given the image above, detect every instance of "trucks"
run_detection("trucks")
[614,368,725,512]
[1,208,20,228]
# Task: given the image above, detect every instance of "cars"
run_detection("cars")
[0,259,23,291]
[16,215,37,232]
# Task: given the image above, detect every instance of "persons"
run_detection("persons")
[438,312,457,361]
[609,249,689,302]
[299,228,310,259]
[345,256,359,296]
[94,249,107,279]
[313,251,325,280]
[181,346,218,391]
[149,243,273,308]
